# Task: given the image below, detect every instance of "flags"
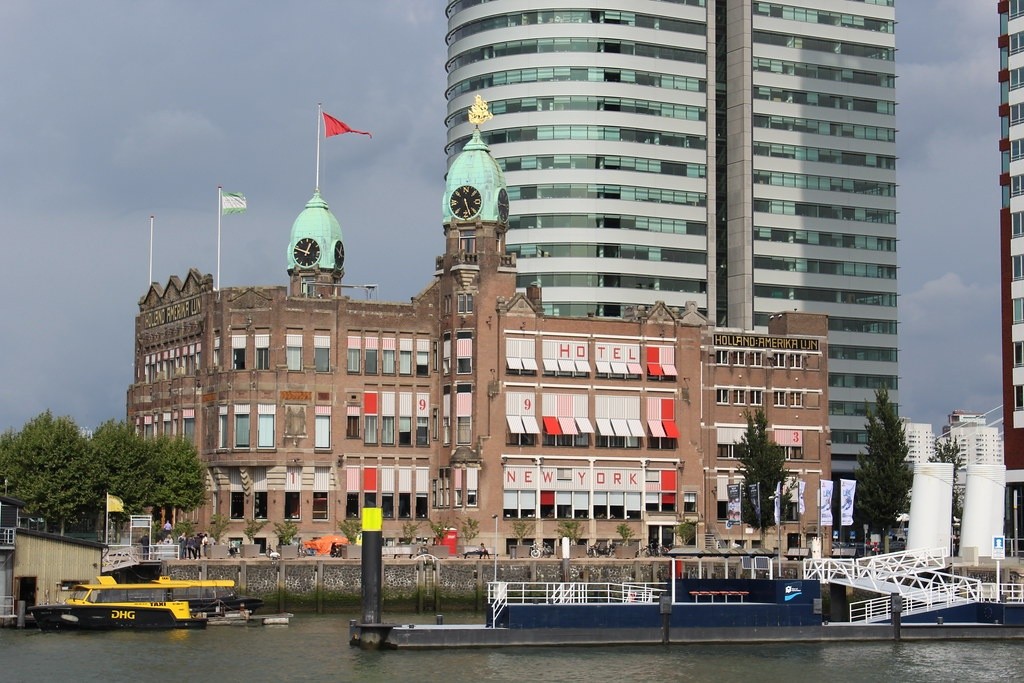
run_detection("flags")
[107,494,123,512]
[220,188,246,215]
[321,111,371,139]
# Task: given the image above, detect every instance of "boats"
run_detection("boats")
[27,605,209,631]
[102,545,162,584]
[120,594,265,618]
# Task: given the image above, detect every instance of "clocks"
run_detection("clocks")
[335,241,345,269]
[294,238,320,266]
[498,189,510,222]
[450,186,481,219]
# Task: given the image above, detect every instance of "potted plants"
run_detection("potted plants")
[205,513,232,559]
[615,521,638,559]
[240,517,271,558]
[273,519,299,559]
[426,516,454,558]
[337,512,361,559]
[554,517,586,558]
[509,517,536,557]
[455,516,480,558]
[397,519,423,557]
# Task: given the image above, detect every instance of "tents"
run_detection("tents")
[303,535,354,555]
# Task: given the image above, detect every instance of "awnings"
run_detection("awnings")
[503,357,681,438]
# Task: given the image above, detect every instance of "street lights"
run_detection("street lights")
[769,482,782,578]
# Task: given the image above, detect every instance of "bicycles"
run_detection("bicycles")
[635,543,670,558]
[530,541,554,559]
[587,542,617,558]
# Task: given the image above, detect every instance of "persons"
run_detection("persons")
[478,542,490,559]
[140,520,209,560]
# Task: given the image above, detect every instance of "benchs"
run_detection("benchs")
[690,591,750,603]
